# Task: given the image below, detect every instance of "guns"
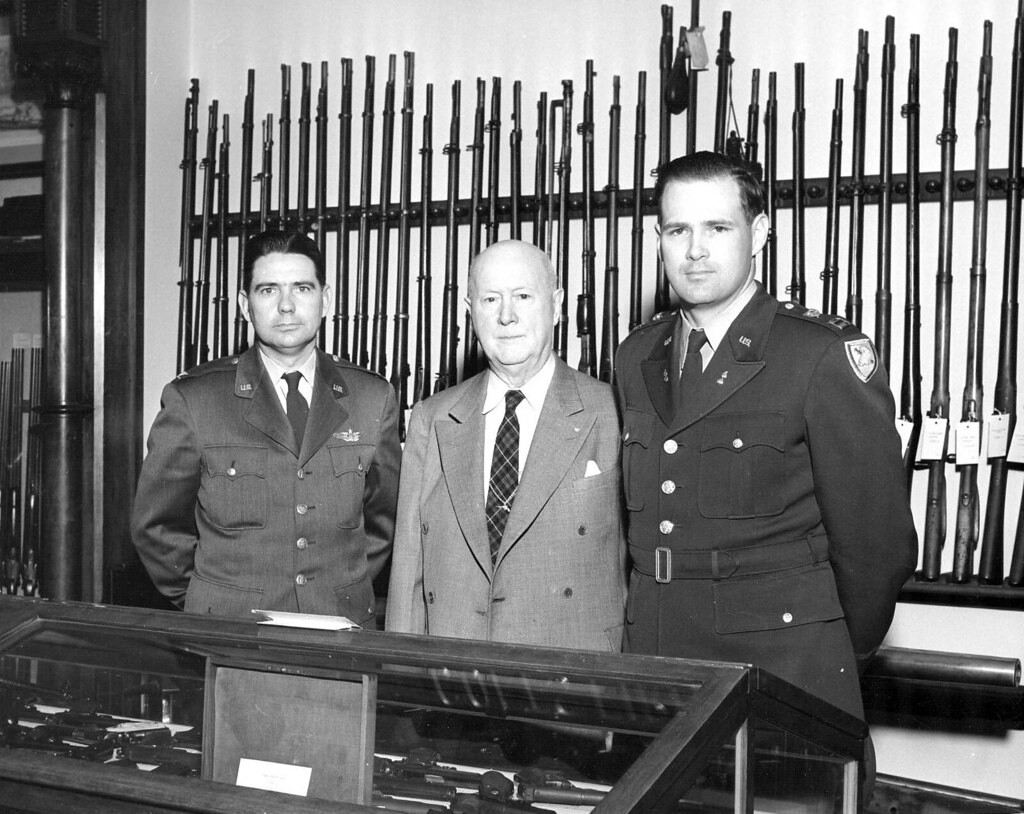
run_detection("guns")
[1,689,609,813]
[170,0,1022,596]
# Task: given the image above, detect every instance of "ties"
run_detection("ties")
[485,389,526,565]
[674,329,707,410]
[281,371,309,456]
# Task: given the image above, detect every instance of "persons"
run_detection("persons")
[616,150,919,720]
[130,227,401,628]
[385,239,627,654]
